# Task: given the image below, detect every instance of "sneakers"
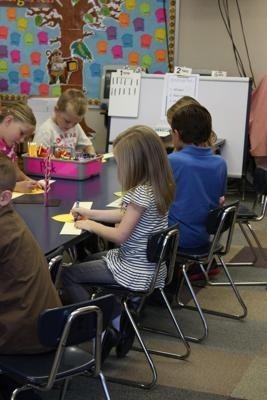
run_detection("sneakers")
[92,327,118,364]
[186,259,219,274]
[113,309,139,357]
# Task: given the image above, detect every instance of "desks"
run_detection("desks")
[11,150,123,262]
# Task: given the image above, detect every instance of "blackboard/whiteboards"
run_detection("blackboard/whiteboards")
[105,72,254,179]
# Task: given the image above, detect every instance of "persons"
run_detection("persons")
[32,87,96,157]
[165,95,220,281]
[0,101,52,194]
[0,154,65,400]
[60,123,176,365]
[148,103,227,321]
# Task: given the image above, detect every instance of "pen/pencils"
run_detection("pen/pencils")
[74,200,80,221]
